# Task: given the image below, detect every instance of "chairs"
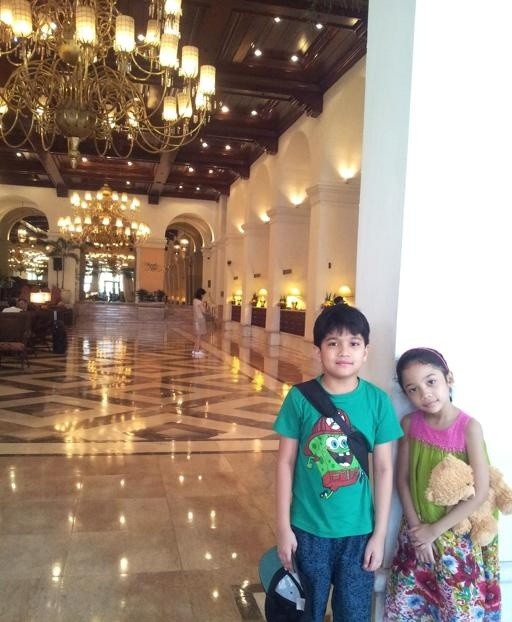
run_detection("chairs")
[1,303,72,340]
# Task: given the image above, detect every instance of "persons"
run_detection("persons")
[191,288,211,355]
[388,347,498,621]
[17,299,36,312]
[1,296,24,313]
[334,296,344,305]
[269,303,399,621]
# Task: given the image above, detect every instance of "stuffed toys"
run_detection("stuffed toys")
[424,452,512,547]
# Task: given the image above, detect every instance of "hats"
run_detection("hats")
[259,546,312,621]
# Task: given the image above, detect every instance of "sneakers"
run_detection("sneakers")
[192,350,204,355]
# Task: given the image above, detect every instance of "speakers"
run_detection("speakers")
[54,258,62,270]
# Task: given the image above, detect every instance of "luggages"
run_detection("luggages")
[52,310,67,353]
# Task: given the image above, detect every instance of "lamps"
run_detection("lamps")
[233,286,353,311]
[57,183,152,268]
[1,1,217,172]
